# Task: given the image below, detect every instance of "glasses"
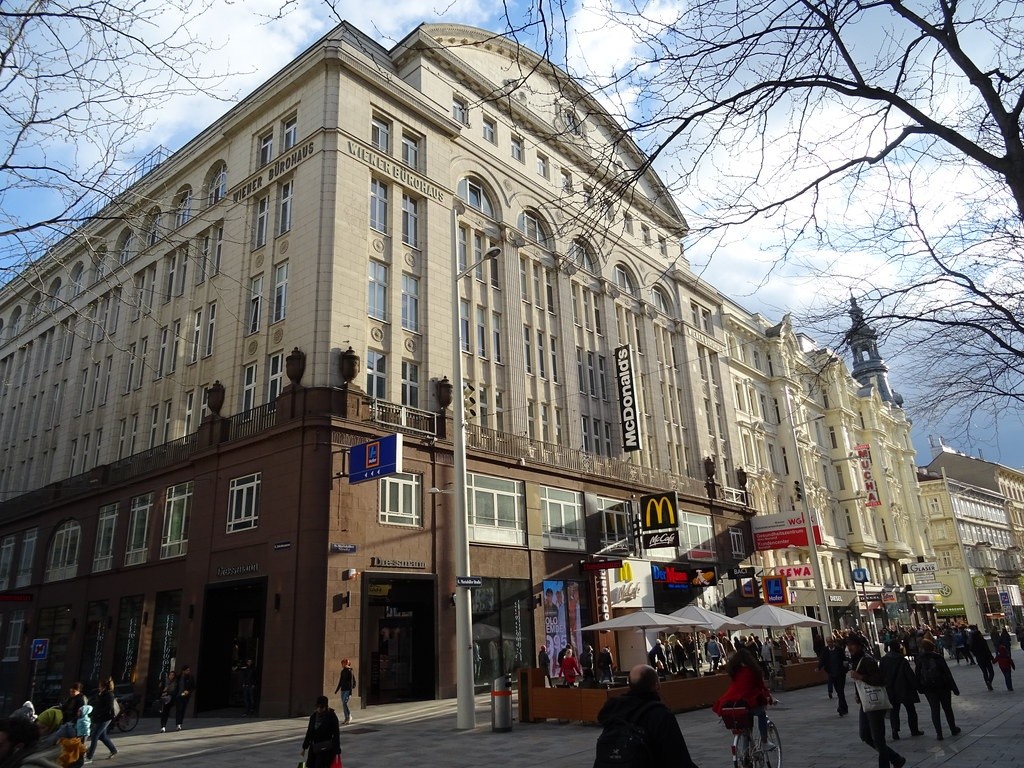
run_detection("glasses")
[316,706,324,708]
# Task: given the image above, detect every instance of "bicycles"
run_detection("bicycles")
[106,697,139,733]
[721,699,784,768]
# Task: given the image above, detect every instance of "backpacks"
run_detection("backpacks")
[594,705,652,768]
[352,674,356,688]
[916,657,944,694]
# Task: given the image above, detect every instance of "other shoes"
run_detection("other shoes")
[240,711,248,718]
[341,721,349,725]
[987,681,993,690]
[1008,688,1014,691]
[951,727,961,736]
[828,692,833,699]
[893,757,906,768]
[837,707,843,716]
[892,728,899,740]
[911,731,924,736]
[937,736,943,740]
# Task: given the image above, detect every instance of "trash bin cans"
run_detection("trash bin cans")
[490,672,514,732]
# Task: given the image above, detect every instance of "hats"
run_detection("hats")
[314,696,328,706]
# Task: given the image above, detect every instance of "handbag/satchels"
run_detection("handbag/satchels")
[313,741,333,753]
[855,679,893,712]
[329,754,342,768]
[297,758,308,768]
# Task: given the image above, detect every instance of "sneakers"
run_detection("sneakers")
[160,727,165,733]
[763,742,776,752]
[176,724,181,730]
[84,757,92,765]
[105,752,119,760]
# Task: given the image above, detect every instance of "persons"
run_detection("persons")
[0,675,119,768]
[815,621,1024,718]
[545,588,565,637]
[592,664,699,768]
[558,645,582,687]
[915,639,961,740]
[160,665,194,732]
[580,645,612,687]
[539,645,555,688]
[649,631,800,675]
[847,633,906,768]
[300,696,341,768]
[712,648,778,768]
[335,658,354,724]
[880,642,924,740]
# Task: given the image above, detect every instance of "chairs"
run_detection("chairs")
[547,658,805,693]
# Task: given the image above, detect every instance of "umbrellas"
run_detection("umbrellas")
[731,602,828,642]
[668,603,752,672]
[576,608,713,664]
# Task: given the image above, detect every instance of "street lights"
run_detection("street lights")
[783,386,833,647]
[450,208,502,729]
[940,467,986,634]
